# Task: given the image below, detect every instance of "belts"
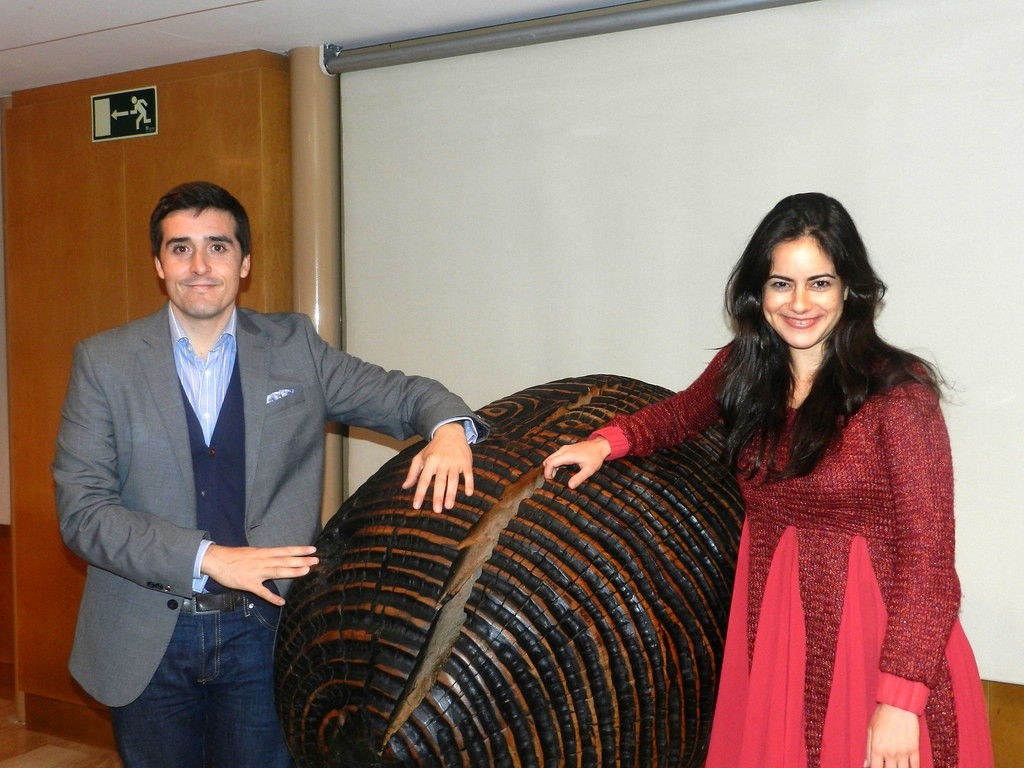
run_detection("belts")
[180,590,262,616]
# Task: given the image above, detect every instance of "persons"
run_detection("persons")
[53,180,493,768]
[544,192,995,768]
